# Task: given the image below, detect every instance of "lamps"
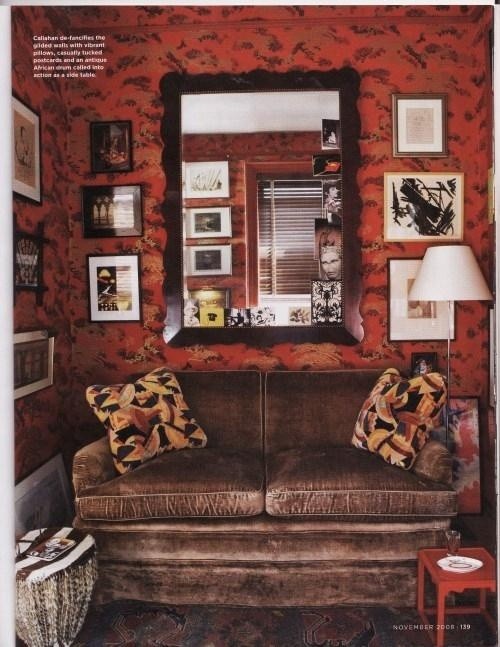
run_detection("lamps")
[409,246,495,389]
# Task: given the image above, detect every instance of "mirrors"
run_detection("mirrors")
[158,66,365,347]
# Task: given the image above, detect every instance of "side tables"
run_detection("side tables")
[417,547,497,646]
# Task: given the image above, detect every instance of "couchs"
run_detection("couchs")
[72,368,460,610]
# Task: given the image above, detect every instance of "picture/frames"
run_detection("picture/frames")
[11,96,43,207]
[448,394,481,516]
[79,184,143,238]
[187,243,233,277]
[392,93,447,158]
[14,454,74,537]
[14,329,55,400]
[189,287,228,313]
[387,257,455,342]
[383,172,464,243]
[87,254,141,323]
[185,205,233,240]
[411,351,437,378]
[89,121,131,173]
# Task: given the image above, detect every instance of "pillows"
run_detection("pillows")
[85,367,207,476]
[351,369,450,473]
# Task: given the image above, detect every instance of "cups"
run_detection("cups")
[445,530,461,556]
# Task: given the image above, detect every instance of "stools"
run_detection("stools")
[15,526,98,647]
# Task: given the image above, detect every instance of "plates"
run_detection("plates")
[436,556,484,574]
